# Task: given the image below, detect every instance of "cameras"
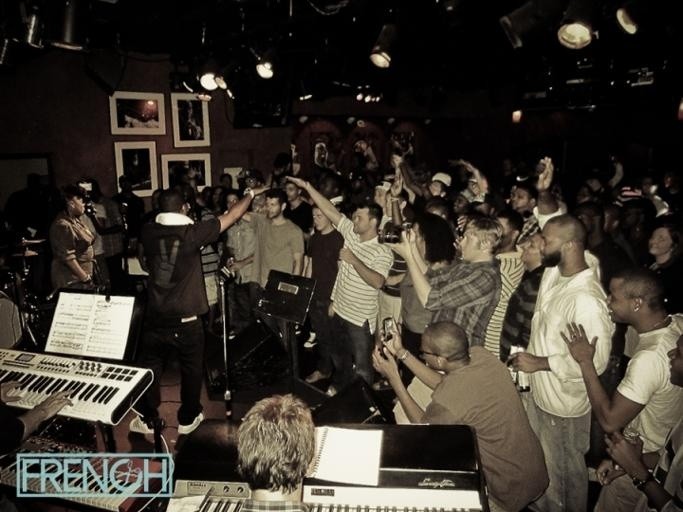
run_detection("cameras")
[375,220,412,244]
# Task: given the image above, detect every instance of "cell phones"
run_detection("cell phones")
[383,317,392,342]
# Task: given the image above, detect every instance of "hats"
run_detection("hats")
[531,189,568,220]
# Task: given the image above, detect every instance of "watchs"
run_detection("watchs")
[633,474,655,491]
[400,351,409,361]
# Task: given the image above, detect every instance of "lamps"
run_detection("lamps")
[52,1,83,52]
[178,71,195,93]
[255,28,296,80]
[369,13,426,71]
[550,2,593,50]
[296,81,312,102]
[0,26,12,66]
[19,2,44,51]
[195,59,243,101]
[501,3,535,50]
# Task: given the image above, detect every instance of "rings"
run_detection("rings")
[571,333,576,338]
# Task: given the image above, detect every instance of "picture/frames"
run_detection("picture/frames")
[170,90,211,148]
[108,89,166,136]
[114,141,157,198]
[160,153,212,193]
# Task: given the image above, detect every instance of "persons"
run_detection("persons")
[391,174,456,404]
[573,202,636,457]
[560,267,683,512]
[373,188,411,391]
[112,176,145,238]
[231,188,304,290]
[138,189,164,272]
[513,164,616,512]
[371,317,549,511]
[612,224,683,388]
[306,203,354,397]
[308,135,683,237]
[304,173,344,348]
[605,334,683,512]
[226,189,259,326]
[452,210,525,360]
[268,153,292,188]
[286,177,393,387]
[76,178,106,292]
[171,180,220,338]
[384,218,502,425]
[184,168,264,215]
[128,182,270,433]
[50,183,96,292]
[0,382,74,456]
[237,395,315,512]
[178,99,198,141]
[283,182,314,240]
[500,234,549,363]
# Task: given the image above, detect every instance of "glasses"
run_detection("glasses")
[418,348,449,362]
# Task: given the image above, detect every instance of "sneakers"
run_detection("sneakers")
[177,413,205,435]
[128,415,157,435]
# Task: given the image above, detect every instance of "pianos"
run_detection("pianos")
[1,436,162,512]
[164,419,490,511]
[0,348,154,426]
